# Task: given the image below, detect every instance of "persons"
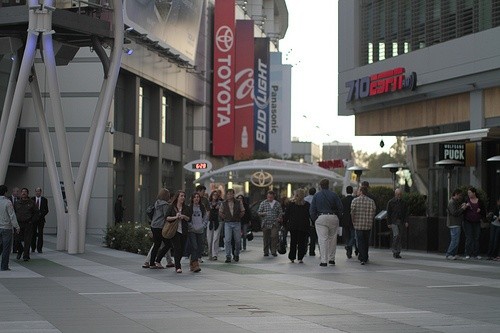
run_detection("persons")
[142,189,175,269]
[0,185,48,271]
[165,190,192,273]
[341,185,355,258]
[487,197,500,261]
[185,185,210,272]
[355,181,371,256]
[207,191,222,260]
[115,194,123,224]
[350,187,376,264]
[257,190,283,256]
[284,188,311,263]
[446,189,467,260]
[461,188,486,259]
[219,189,245,262]
[386,188,409,259]
[303,187,316,256]
[309,179,342,267]
[282,196,289,247]
[237,195,250,252]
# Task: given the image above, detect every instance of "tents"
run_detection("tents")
[193,157,343,187]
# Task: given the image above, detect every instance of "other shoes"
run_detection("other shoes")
[345,245,352,258]
[17,254,21,259]
[234,255,239,262]
[224,256,231,263]
[329,261,335,264]
[446,255,457,260]
[320,263,327,266]
[466,255,470,259]
[476,256,482,259]
[141,264,148,268]
[166,263,175,267]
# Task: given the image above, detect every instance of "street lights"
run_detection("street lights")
[347,166,370,190]
[435,160,464,226]
[382,163,408,192]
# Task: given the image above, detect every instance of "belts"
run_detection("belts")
[318,212,337,215]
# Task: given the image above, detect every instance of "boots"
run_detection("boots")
[190,260,194,271]
[192,261,201,272]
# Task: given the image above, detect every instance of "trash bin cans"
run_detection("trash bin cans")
[408,216,439,250]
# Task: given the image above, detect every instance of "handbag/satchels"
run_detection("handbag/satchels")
[278,241,286,255]
[247,233,254,241]
[146,206,155,220]
[161,219,179,240]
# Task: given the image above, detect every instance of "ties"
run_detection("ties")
[36,199,39,209]
[14,198,16,203]
